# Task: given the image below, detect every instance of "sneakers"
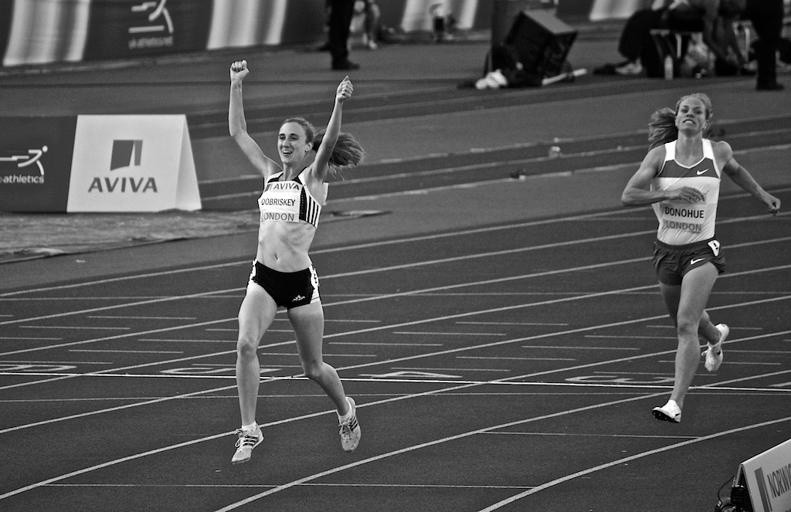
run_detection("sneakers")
[336,396,362,453]
[700,323,729,373]
[330,60,362,72]
[230,422,264,465]
[651,400,682,424]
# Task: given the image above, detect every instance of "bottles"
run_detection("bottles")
[664,52,673,80]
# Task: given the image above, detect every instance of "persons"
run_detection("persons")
[428,0,467,45]
[344,0,381,53]
[614,0,791,91]
[328,0,362,73]
[225,58,363,465]
[619,88,782,422]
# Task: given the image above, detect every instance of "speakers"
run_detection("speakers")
[504,8,578,90]
[617,8,706,79]
